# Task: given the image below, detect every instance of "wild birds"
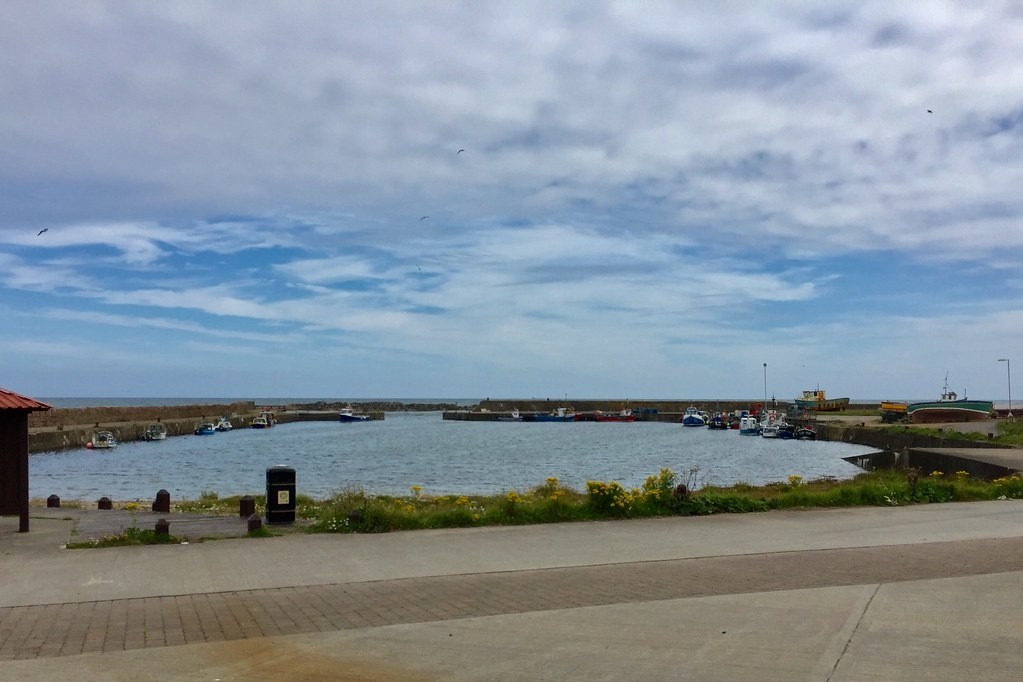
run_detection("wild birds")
[456,149,464,155]
[419,216,430,222]
[927,109,933,113]
[36,228,49,236]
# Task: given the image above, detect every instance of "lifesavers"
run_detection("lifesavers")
[144,432,149,436]
[567,411,570,415]
[769,416,775,420]
[761,411,765,415]
[499,403,503,407]
[219,426,223,430]
[141,435,146,439]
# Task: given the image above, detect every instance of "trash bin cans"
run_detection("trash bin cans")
[264,465,296,525]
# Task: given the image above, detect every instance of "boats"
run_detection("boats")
[197,423,217,435]
[681,403,708,426]
[905,370,995,425]
[142,421,167,443]
[497,405,525,422]
[876,401,909,420]
[248,411,277,429]
[793,382,851,413]
[631,406,660,422]
[705,363,797,438]
[86,428,118,448]
[796,423,817,441]
[339,402,370,422]
[215,420,233,430]
[529,407,584,422]
[596,395,638,422]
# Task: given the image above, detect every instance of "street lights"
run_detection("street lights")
[997,359,1015,416]
